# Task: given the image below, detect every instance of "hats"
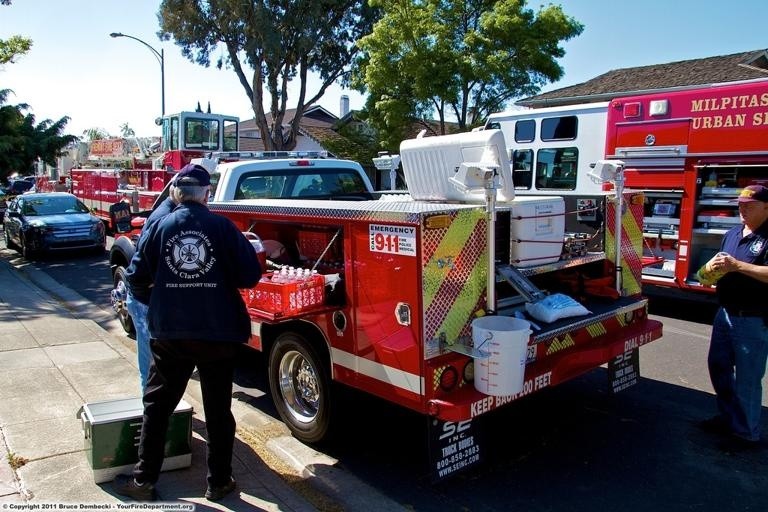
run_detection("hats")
[729,184,768,203]
[174,163,210,186]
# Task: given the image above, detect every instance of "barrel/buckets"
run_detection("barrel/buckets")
[470,314,532,397]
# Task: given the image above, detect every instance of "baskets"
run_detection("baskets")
[237,271,326,323]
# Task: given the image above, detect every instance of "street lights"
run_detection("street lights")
[109,29,165,115]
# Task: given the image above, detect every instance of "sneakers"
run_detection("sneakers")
[112,474,156,501]
[699,415,730,433]
[714,433,761,449]
[205,474,236,500]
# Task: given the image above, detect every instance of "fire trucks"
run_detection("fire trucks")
[65,75,768,494]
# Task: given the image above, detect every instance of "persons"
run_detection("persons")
[123,184,180,397]
[112,161,261,500]
[699,184,767,451]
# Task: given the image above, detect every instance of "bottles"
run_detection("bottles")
[257,259,345,310]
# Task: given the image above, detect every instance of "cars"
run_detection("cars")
[0,172,44,206]
[2,190,110,263]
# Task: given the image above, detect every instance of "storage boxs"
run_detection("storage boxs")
[76,397,194,485]
